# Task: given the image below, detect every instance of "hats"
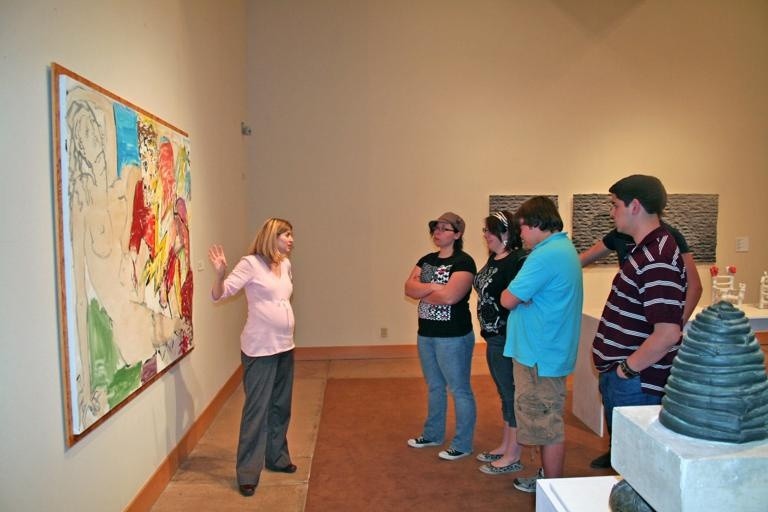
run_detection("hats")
[429,212,465,234]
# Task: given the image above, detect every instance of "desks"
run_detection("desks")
[685,303,768,336]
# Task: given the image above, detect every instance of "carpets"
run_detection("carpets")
[302,372,620,512]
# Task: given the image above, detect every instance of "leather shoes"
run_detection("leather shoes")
[240,484,256,496]
[265,463,296,473]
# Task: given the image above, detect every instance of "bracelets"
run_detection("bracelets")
[620,359,639,378]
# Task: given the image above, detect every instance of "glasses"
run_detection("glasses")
[432,226,457,233]
[483,228,493,234]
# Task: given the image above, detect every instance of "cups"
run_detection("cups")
[712,276,744,309]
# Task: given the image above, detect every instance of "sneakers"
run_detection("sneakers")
[592,453,611,468]
[438,447,471,460]
[476,450,504,462]
[478,460,524,474]
[407,435,440,448]
[513,467,544,492]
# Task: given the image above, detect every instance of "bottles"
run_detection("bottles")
[759,271,768,309]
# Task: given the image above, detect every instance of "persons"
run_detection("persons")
[404,212,476,461]
[472,209,530,476]
[208,217,297,496]
[579,178,703,469]
[501,195,583,494]
[66,86,179,429]
[591,175,688,436]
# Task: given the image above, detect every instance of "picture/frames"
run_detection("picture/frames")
[50,61,195,450]
